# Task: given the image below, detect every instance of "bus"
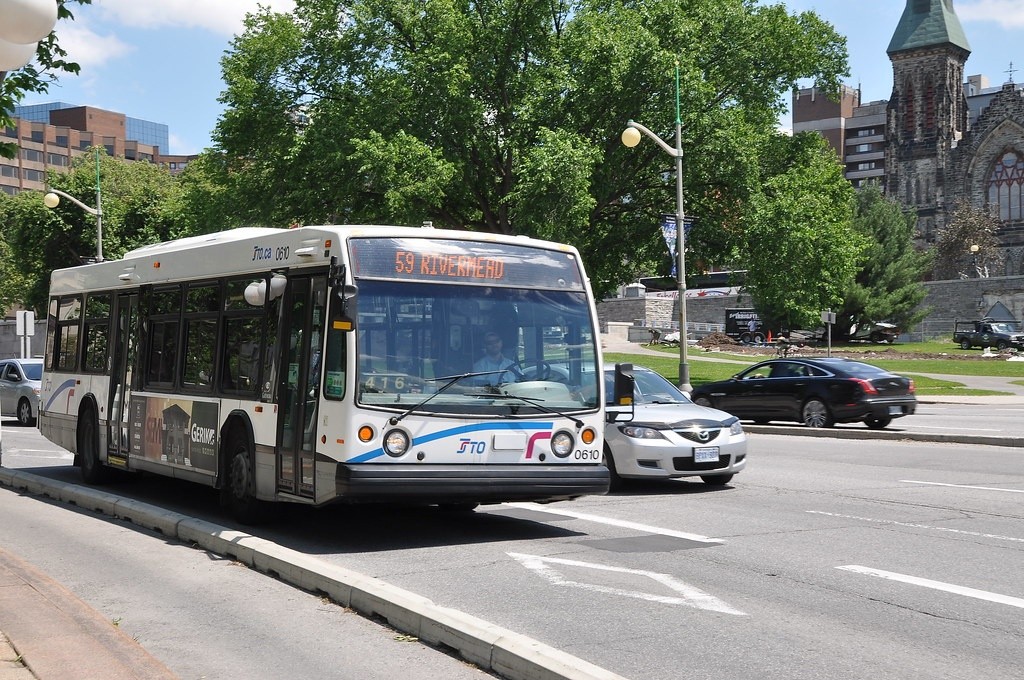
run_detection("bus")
[638,270,756,298]
[35,221,637,527]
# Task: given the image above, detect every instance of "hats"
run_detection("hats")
[484,332,497,340]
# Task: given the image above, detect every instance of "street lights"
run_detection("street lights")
[44,144,104,263]
[621,60,694,395]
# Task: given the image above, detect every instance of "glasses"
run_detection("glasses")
[487,339,500,344]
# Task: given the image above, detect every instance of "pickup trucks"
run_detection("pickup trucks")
[952,316,1024,352]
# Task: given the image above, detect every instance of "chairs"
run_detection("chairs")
[442,352,476,386]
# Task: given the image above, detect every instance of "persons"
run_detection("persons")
[648,329,662,345]
[472,332,524,386]
[776,333,790,358]
[748,318,757,341]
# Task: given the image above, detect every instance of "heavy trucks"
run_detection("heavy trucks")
[724,308,828,343]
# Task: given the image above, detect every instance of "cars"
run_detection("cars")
[521,362,750,493]
[690,356,917,430]
[849,313,900,344]
[0,359,43,427]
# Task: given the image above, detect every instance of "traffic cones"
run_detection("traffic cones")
[767,329,772,342]
